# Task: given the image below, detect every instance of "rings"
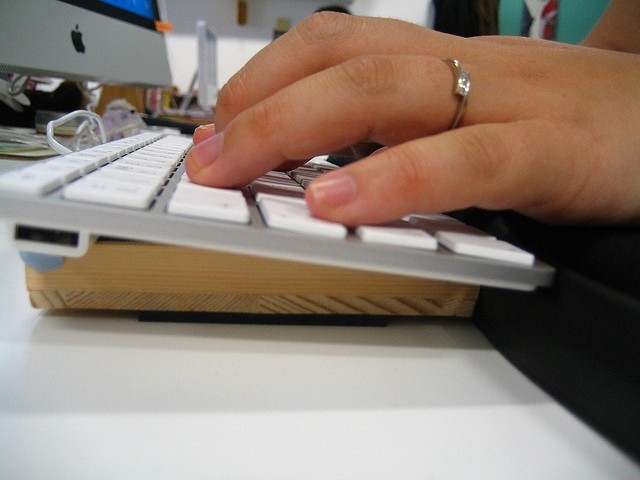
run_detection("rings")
[442,57,471,129]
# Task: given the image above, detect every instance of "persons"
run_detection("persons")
[184,0,638,462]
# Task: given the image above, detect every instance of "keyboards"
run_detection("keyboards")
[2,127,556,326]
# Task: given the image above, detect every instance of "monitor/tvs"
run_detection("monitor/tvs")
[179,19,218,118]
[0,0,174,89]
